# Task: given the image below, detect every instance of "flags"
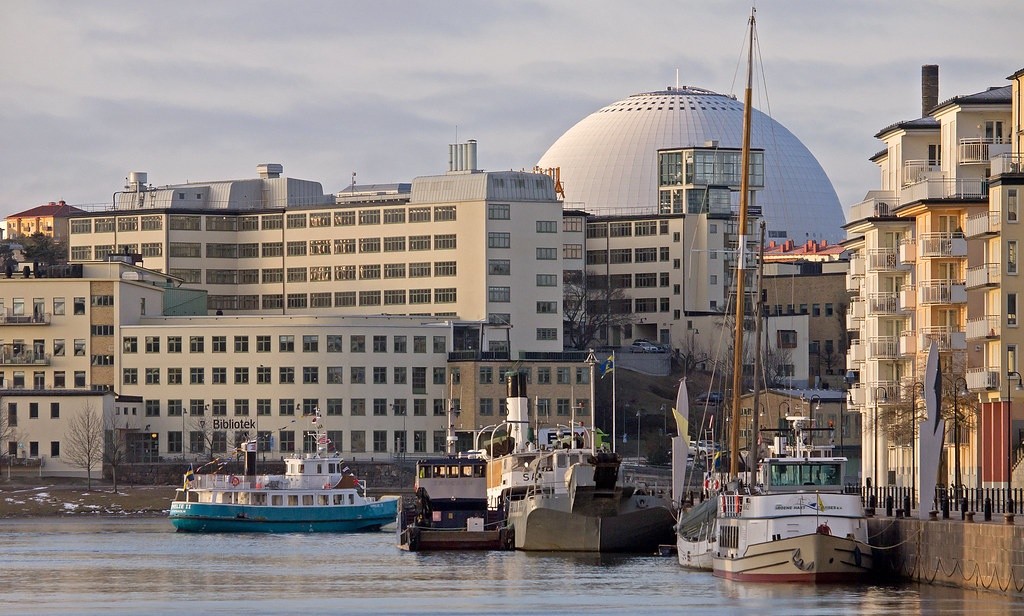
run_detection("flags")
[672,408,690,449]
[184,464,195,495]
[712,442,724,471]
[818,494,825,512]
[600,354,616,379]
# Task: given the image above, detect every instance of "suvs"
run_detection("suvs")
[695,391,724,403]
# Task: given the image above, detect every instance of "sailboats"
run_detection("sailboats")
[677,1,874,583]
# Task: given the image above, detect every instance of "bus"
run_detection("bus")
[621,457,647,466]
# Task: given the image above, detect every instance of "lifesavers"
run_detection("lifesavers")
[705,477,720,492]
[231,478,240,487]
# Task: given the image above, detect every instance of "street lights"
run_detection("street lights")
[476,424,497,451]
[623,399,628,459]
[779,402,790,457]
[584,349,601,456]
[557,420,590,448]
[809,394,822,485]
[874,387,888,514]
[954,376,970,512]
[635,409,640,469]
[660,404,667,451]
[912,381,924,510]
[1006,371,1024,524]
[746,402,766,487]
[840,390,854,456]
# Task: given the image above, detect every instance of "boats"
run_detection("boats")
[169,404,401,533]
[394,347,680,554]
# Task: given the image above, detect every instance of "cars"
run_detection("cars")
[688,439,722,456]
[747,437,774,451]
[630,339,665,353]
